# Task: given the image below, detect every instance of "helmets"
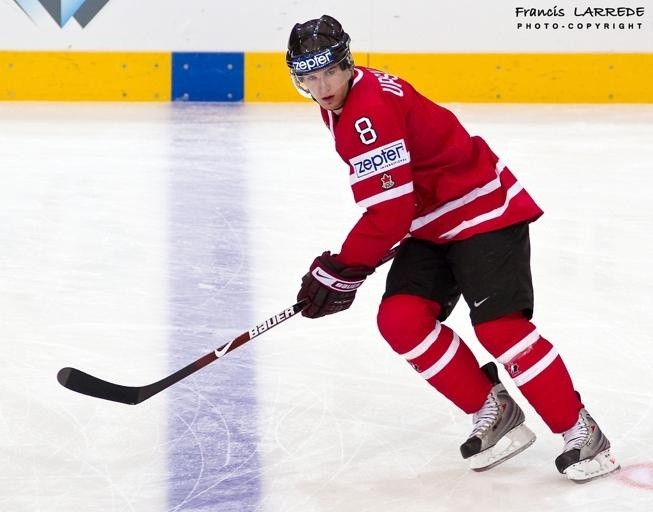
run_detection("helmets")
[286,15,354,99]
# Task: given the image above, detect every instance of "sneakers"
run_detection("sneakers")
[460,381,525,458]
[555,408,610,474]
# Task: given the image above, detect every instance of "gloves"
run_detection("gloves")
[297,251,375,317]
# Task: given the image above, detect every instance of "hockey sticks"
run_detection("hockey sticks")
[57,246,396,405]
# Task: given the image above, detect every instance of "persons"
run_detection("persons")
[286,15,612,473]
